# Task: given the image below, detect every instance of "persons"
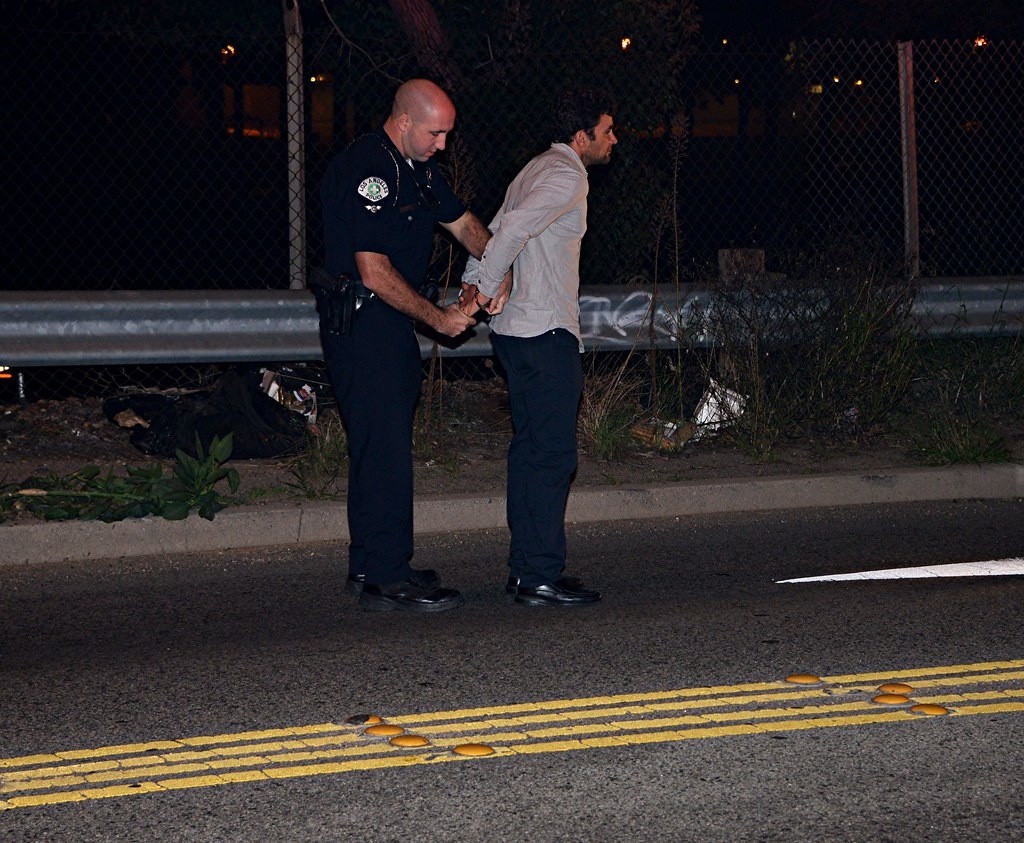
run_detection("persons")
[452,87,617,610]
[309,79,512,613]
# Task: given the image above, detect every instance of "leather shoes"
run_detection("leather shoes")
[358,582,465,613]
[514,582,602,607]
[346,568,440,597]
[505,574,585,595]
